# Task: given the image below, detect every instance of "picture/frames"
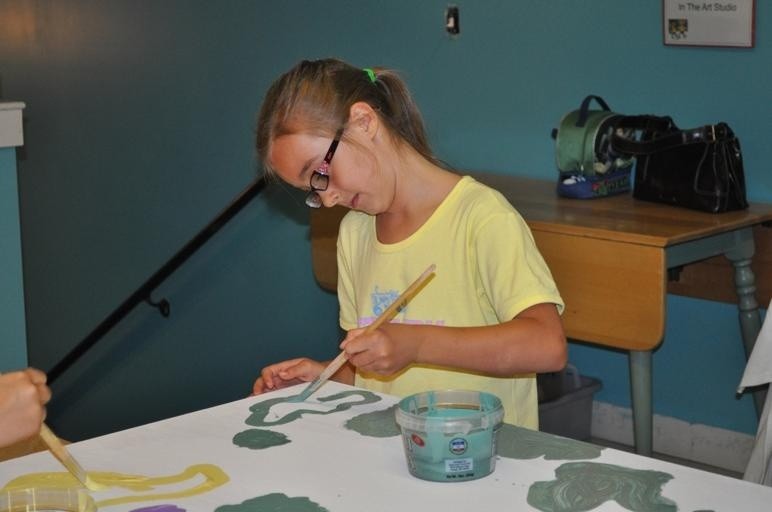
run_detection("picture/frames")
[661,0,757,50]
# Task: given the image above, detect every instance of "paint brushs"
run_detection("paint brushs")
[302,264,437,401]
[39,422,102,493]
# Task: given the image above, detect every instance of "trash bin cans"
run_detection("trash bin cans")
[537,374,602,441]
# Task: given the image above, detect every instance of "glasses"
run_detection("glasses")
[305,106,383,208]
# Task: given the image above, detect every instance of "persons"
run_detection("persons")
[0,367,53,451]
[241,55,571,431]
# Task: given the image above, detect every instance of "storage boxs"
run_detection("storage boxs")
[533,364,601,439]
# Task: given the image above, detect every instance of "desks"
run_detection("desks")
[303,171,771,461]
[2,377,771,512]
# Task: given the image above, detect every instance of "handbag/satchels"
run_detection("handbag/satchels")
[556,94,636,176]
[633,117,748,213]
